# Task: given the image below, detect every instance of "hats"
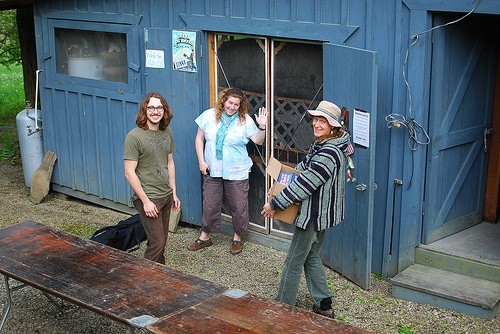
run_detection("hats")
[306,100,342,132]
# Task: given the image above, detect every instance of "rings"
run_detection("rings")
[266,212,268,215]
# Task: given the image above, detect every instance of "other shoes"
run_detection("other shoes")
[187,237,213,251]
[230,239,243,254]
[313,303,334,318]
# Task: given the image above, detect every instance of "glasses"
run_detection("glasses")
[146,106,164,112]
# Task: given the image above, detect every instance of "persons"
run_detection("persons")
[123,93,180,265]
[187,88,267,255]
[261,100,350,319]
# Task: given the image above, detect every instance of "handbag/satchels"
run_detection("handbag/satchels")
[89,214,148,254]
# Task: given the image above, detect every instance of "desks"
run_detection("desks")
[146,288,377,334]
[0,221,230,334]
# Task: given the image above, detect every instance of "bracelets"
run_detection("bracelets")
[258,126,266,131]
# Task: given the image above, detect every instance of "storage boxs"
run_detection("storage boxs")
[267,157,303,224]
